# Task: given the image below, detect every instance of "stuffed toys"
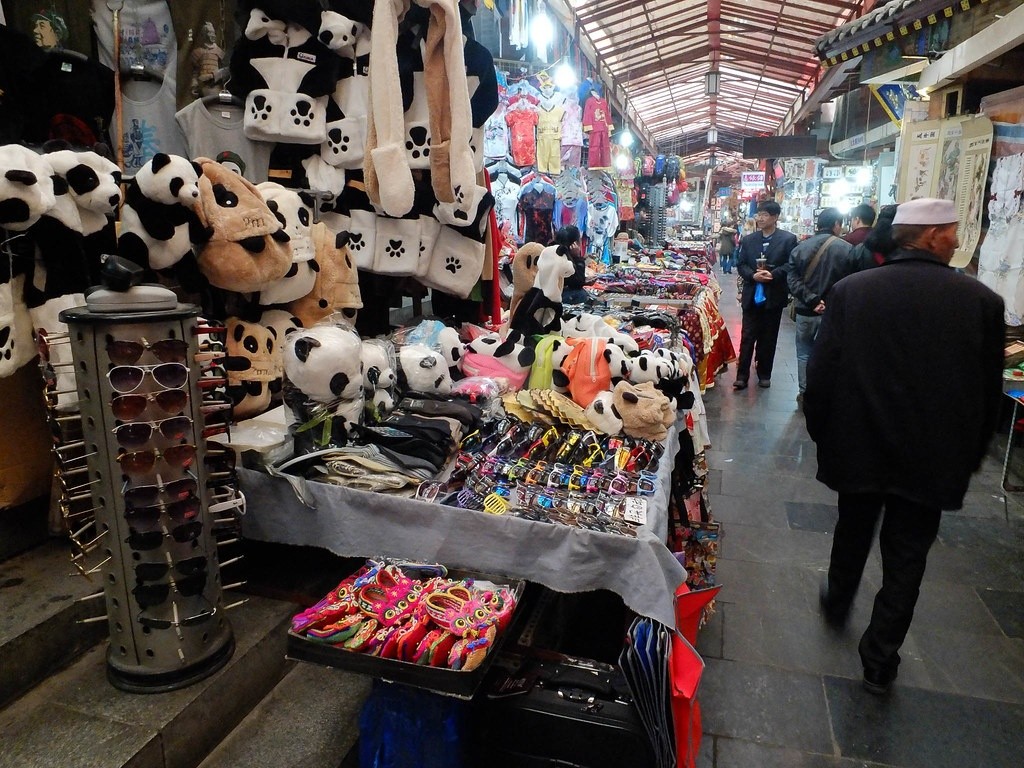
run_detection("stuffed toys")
[279,318,697,488]
[1,143,217,302]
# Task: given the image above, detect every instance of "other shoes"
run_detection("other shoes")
[864,673,890,695]
[820,584,849,632]
[733,381,748,387]
[759,380,770,386]
[797,394,803,402]
[292,560,515,670]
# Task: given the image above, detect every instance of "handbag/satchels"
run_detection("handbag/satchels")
[617,577,725,768]
[789,299,796,322]
[355,687,462,768]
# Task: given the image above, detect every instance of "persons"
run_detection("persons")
[840,203,876,246]
[184,21,232,96]
[733,201,798,388]
[663,212,763,274]
[786,208,858,403]
[853,204,902,272]
[802,198,1006,694]
[555,224,588,306]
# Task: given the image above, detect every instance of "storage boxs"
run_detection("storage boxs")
[287,569,528,698]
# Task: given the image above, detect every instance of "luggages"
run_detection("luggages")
[488,647,655,768]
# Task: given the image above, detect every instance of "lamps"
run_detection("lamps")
[615,95,629,170]
[707,123,719,145]
[619,71,632,146]
[709,155,716,167]
[704,62,722,95]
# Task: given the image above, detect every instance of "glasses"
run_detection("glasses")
[36,320,250,630]
[414,415,665,538]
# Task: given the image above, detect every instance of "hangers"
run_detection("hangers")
[115,41,164,86]
[38,27,91,69]
[517,73,609,208]
[198,70,248,111]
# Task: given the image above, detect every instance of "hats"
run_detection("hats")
[890,198,959,225]
